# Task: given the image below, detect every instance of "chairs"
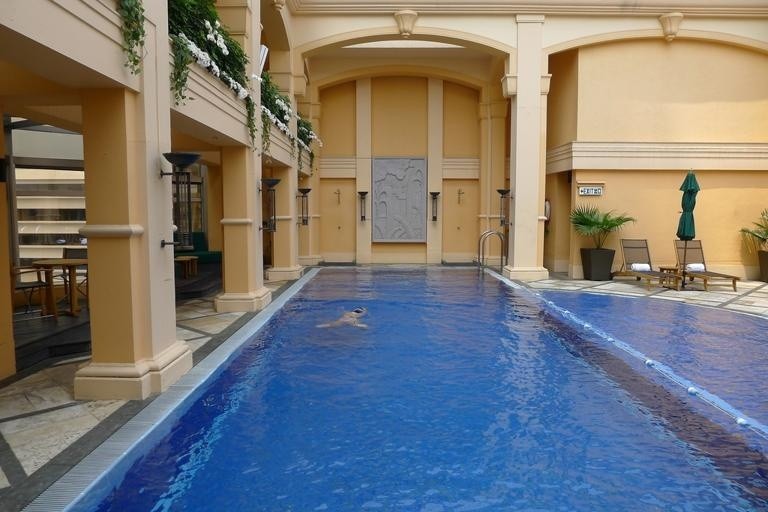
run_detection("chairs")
[672,238,739,293]
[608,238,684,293]
[9,260,55,323]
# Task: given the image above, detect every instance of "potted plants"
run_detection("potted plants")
[566,197,637,281]
[738,208,767,282]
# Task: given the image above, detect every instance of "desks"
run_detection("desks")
[31,256,88,317]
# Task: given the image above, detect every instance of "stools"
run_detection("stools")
[656,263,681,286]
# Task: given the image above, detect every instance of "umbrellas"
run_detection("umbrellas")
[673,166,702,291]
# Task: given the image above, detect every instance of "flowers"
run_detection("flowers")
[182,11,326,157]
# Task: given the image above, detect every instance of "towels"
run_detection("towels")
[685,262,706,272]
[631,261,651,273]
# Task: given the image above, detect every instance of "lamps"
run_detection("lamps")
[356,191,370,220]
[428,190,442,220]
[295,187,312,225]
[158,150,199,252]
[258,177,285,234]
[496,188,510,226]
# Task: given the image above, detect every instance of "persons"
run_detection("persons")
[311,303,369,331]
